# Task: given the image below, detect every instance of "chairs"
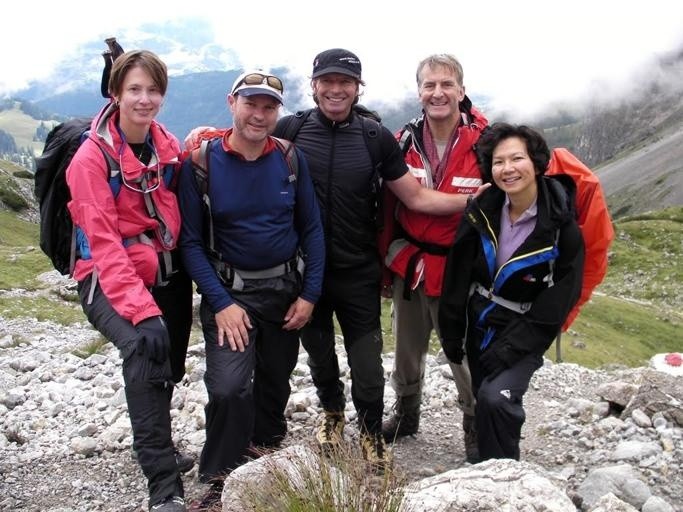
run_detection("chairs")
[383,414,418,442]
[359,431,392,472]
[173,449,198,475]
[150,496,187,511]
[463,432,479,463]
[193,478,223,512]
[317,410,346,452]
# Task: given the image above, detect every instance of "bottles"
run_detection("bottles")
[312,49,361,80]
[230,70,283,107]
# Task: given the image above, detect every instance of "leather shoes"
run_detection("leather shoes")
[442,338,465,365]
[480,350,505,383]
[135,315,172,363]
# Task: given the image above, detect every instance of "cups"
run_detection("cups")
[231,73,283,95]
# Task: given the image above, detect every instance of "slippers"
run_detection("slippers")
[33,118,173,276]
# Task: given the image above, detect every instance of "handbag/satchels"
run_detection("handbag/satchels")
[466,193,474,205]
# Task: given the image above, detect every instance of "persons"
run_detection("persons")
[436,121,589,465]
[173,69,328,512]
[181,46,492,473]
[381,53,492,466]
[61,49,197,512]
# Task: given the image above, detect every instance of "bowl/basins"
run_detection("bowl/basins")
[138,141,148,161]
